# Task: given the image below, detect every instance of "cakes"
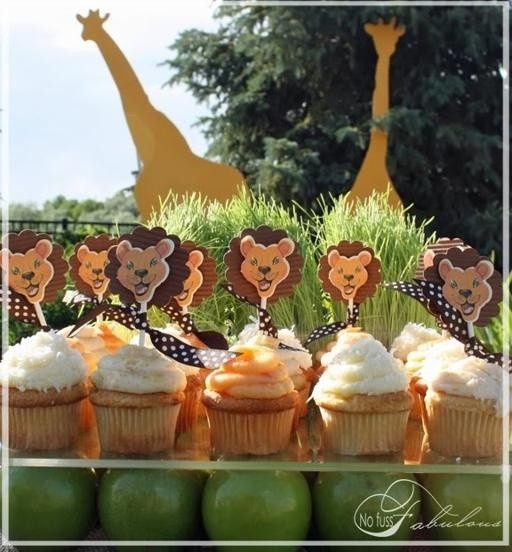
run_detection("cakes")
[0,225,512,458]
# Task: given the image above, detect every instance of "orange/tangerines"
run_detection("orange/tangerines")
[0,467,512,552]
